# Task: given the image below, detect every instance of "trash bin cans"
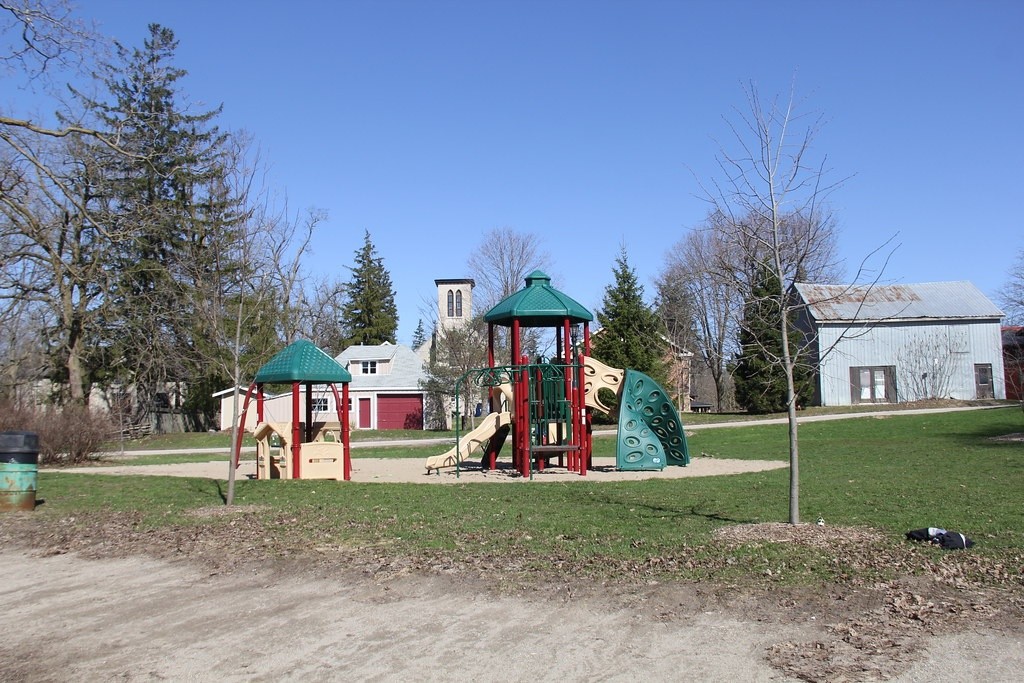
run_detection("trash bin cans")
[0,430,41,513]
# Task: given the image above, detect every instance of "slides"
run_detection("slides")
[424,411,511,470]
